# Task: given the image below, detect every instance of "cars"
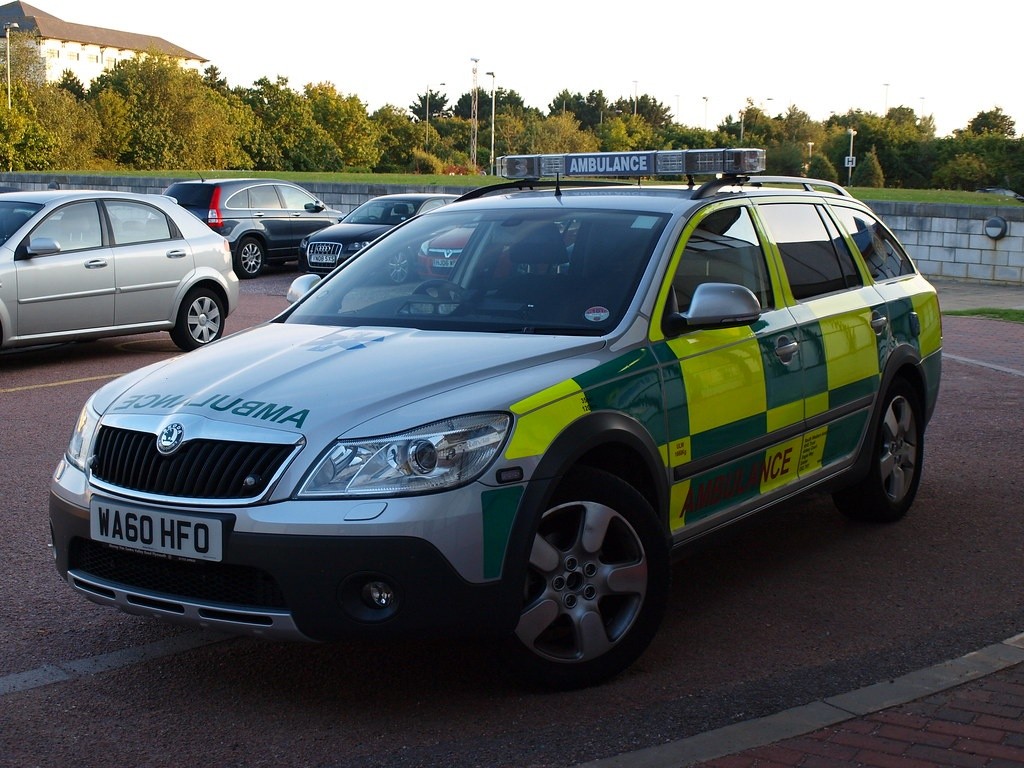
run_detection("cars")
[156,172,347,278]
[973,187,1024,211]
[300,193,465,280]
[417,212,586,298]
[0,190,240,366]
[46,146,945,692]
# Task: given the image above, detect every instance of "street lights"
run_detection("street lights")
[426,82,446,174]
[846,129,859,186]
[807,142,815,177]
[2,21,21,173]
[703,95,709,151]
[748,98,774,147]
[485,71,496,176]
